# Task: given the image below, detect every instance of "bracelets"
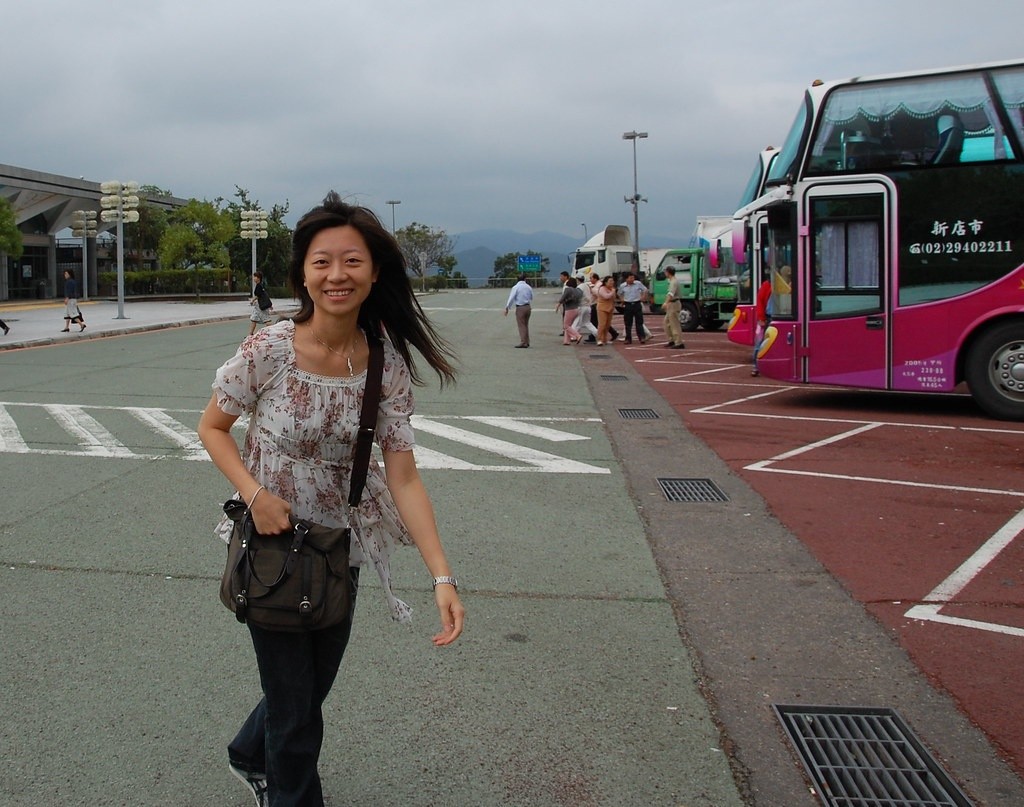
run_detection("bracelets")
[246,486,265,510]
[433,576,458,590]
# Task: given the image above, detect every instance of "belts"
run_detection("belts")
[669,298,680,302]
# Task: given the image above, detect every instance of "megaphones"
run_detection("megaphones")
[641,197,648,203]
[624,195,633,203]
[634,194,642,202]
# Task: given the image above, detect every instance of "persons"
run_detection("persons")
[250,273,273,335]
[662,266,685,349]
[197,190,464,807]
[556,271,655,345]
[61,269,86,332]
[504,273,533,348]
[0,319,10,336]
[750,265,791,377]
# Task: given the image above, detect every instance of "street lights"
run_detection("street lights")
[386,200,401,235]
[240,211,268,297]
[100,181,139,319]
[581,222,587,243]
[72,209,98,299]
[623,130,648,276]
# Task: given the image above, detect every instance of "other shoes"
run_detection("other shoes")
[623,340,632,344]
[229,763,268,807]
[4,326,10,335]
[664,342,685,349]
[515,344,529,348]
[558,330,619,345]
[645,333,653,341]
[639,338,645,343]
[618,338,625,342]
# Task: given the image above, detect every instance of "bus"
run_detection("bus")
[710,56,1024,421]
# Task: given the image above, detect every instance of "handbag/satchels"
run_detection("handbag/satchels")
[71,312,84,324]
[217,501,352,632]
[259,290,273,310]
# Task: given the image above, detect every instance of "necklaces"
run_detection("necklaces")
[307,322,359,376]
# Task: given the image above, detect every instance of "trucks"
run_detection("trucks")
[566,216,734,316]
[647,248,740,331]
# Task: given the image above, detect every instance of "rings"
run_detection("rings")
[450,623,454,627]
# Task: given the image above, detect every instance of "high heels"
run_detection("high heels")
[61,328,69,332]
[79,325,86,332]
